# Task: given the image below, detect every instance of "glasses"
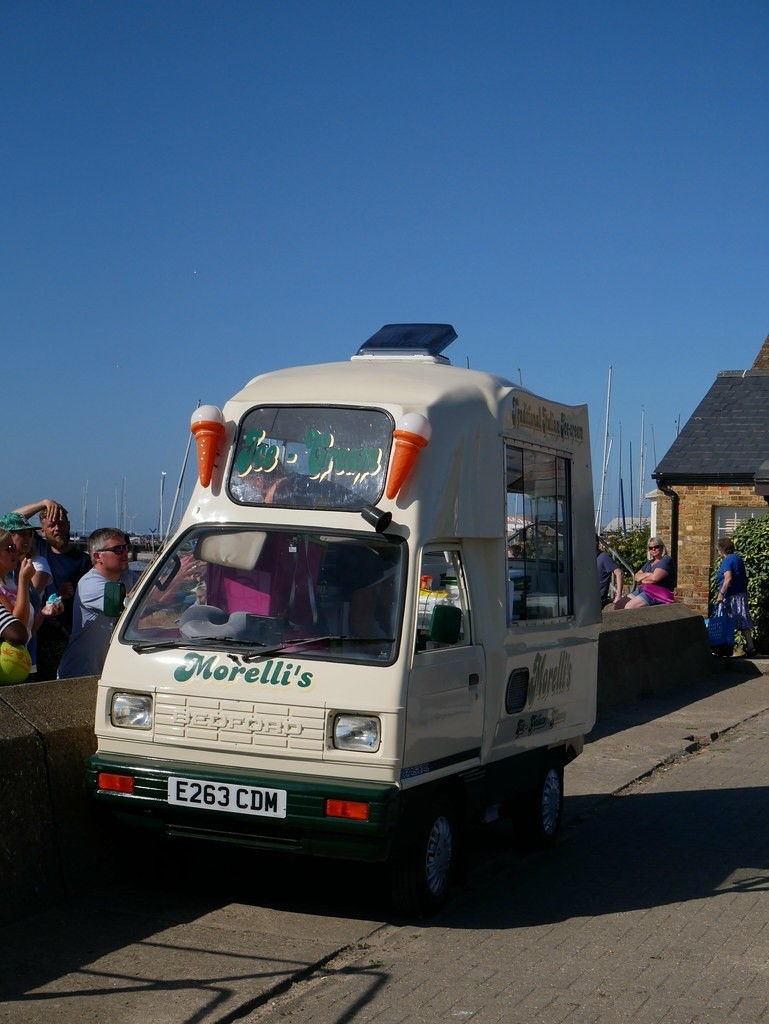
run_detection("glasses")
[1,544,17,554]
[648,545,662,550]
[95,543,130,555]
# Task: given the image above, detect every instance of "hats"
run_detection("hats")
[0,513,43,532]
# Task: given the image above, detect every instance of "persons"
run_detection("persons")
[55,528,207,679]
[595,533,624,604]
[614,537,677,610]
[0,500,95,681]
[716,537,757,658]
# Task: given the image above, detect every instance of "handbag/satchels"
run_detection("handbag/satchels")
[707,601,735,645]
[643,582,675,605]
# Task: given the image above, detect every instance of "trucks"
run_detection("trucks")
[86,321,601,919]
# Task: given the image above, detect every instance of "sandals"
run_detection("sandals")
[744,647,757,658]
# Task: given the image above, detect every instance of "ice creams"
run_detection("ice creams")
[384,413,434,499]
[190,404,224,488]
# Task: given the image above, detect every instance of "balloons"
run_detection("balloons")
[0,641,32,685]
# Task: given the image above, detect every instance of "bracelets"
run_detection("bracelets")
[719,591,724,595]
